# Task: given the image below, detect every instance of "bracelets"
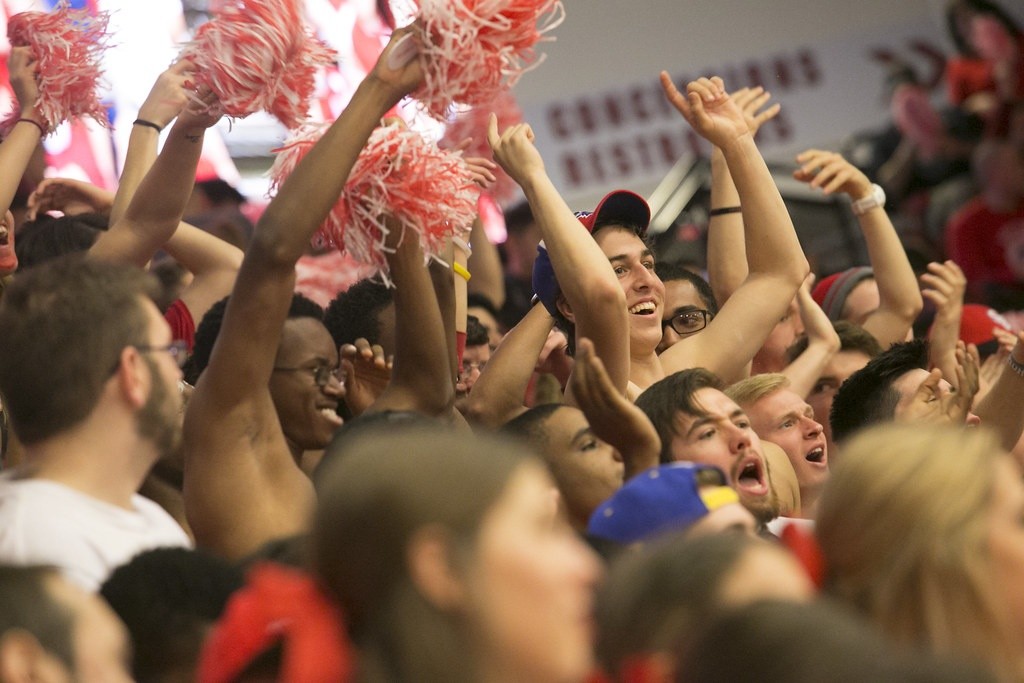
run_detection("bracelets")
[453,236,472,258]
[454,263,471,282]
[709,206,744,216]
[16,119,44,137]
[132,118,162,133]
[1008,354,1024,378]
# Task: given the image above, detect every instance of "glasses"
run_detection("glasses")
[105,339,187,380]
[662,308,714,334]
[272,364,347,387]
[459,360,486,375]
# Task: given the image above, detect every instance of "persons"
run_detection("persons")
[0,0,1024,683]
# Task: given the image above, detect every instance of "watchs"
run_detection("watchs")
[853,183,885,216]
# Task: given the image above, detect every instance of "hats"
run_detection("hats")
[586,462,755,572]
[812,266,875,322]
[532,189,651,316]
[927,305,1015,361]
